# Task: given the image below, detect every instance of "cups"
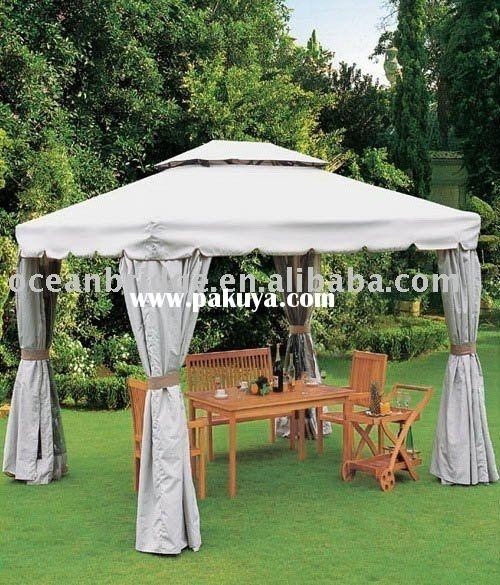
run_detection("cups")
[210,368,328,400]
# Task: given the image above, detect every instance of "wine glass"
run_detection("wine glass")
[394,393,412,415]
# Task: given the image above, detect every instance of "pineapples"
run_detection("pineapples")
[368,382,381,415]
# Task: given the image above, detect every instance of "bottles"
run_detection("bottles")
[286,352,298,392]
[378,396,390,415]
[396,423,416,459]
[272,343,283,393]
